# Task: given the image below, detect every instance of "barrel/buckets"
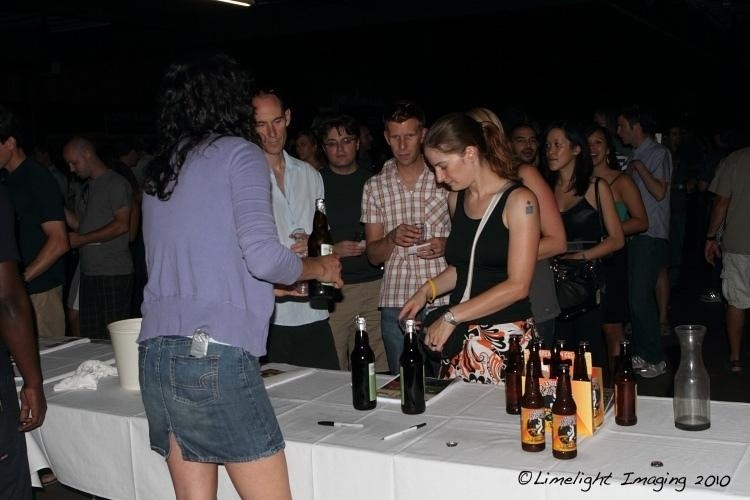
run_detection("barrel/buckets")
[106,317,143,391]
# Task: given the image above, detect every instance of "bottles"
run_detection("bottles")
[349,314,380,411]
[307,195,339,308]
[673,323,713,431]
[504,328,638,461]
[398,317,427,416]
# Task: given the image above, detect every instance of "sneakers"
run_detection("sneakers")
[632,354,647,368]
[658,321,670,336]
[638,358,668,378]
[700,288,721,304]
[724,358,744,375]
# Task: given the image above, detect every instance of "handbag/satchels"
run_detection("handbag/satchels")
[420,304,468,363]
[552,263,601,313]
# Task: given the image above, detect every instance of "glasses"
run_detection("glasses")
[324,138,356,145]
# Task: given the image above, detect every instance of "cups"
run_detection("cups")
[106,318,150,392]
[411,221,428,245]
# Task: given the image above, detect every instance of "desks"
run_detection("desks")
[10,336,750,500]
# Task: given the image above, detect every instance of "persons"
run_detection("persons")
[35,90,748,378]
[139,53,343,500]
[1,112,71,487]
[0,181,47,498]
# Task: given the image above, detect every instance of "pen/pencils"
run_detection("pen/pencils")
[318,421,364,428]
[381,423,426,440]
